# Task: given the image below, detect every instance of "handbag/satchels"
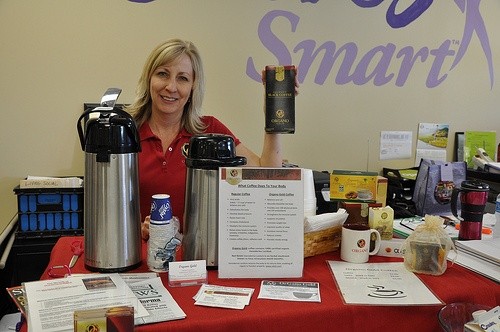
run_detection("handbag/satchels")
[412,158,469,217]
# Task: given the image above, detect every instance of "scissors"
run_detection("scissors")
[69,240,85,268]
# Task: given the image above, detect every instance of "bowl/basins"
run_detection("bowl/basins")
[438,303,494,332]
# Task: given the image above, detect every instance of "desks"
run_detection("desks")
[18,232,500,332]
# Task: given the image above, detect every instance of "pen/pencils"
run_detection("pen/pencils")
[204,290,249,296]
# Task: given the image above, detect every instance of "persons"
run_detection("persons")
[122,39,299,242]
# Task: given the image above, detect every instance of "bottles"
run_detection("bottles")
[493,193,500,240]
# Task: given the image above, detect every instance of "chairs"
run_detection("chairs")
[383,168,414,210]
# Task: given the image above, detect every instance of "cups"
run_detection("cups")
[341,224,381,264]
[451,181,490,241]
[302,169,317,218]
[358,190,373,199]
[147,194,177,273]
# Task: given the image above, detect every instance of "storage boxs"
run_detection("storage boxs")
[329,170,389,227]
[12,176,84,232]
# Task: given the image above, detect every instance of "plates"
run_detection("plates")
[357,196,374,200]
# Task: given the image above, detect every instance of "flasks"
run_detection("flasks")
[76,106,141,273]
[182,133,247,270]
